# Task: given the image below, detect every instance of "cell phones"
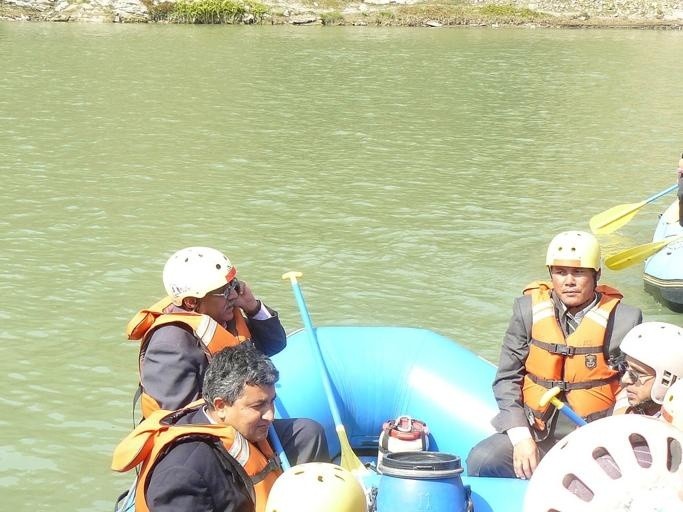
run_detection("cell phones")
[230,278,240,291]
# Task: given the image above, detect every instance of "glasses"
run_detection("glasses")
[206,278,237,300]
[622,359,655,381]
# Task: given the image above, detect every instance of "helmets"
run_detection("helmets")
[161,246,236,307]
[618,321,683,405]
[545,230,602,271]
[658,378,683,428]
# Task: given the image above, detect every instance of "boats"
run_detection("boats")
[642,198,683,306]
[122,324,530,512]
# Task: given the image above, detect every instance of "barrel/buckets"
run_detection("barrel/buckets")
[378,417,430,474]
[376,451,467,511]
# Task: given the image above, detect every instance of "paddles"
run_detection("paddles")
[281,271,370,472]
[589,183,679,238]
[604,233,682,272]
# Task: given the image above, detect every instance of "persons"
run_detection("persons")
[125,246,330,468]
[618,321,683,428]
[677,153,683,227]
[111,342,280,512]
[464,231,644,479]
